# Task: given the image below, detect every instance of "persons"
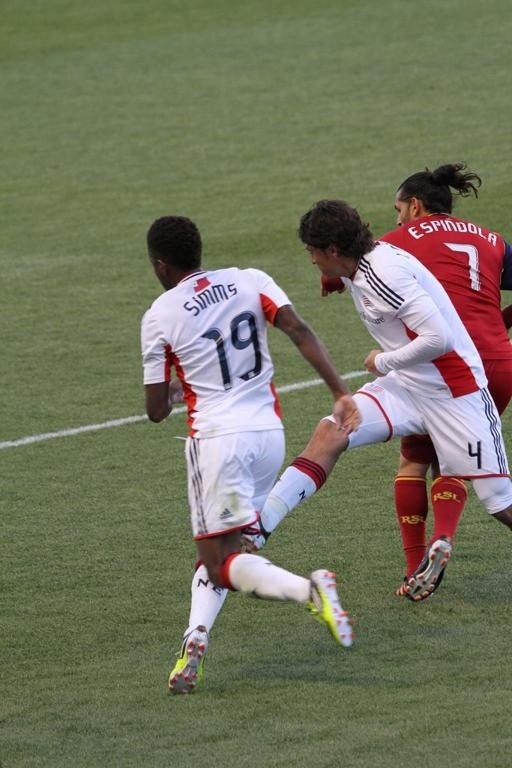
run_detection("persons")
[137,215,366,699]
[320,161,512,601]
[240,200,512,552]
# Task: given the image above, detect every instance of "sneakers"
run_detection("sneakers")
[238,510,271,554]
[395,535,453,602]
[305,569,356,648]
[168,625,209,695]
